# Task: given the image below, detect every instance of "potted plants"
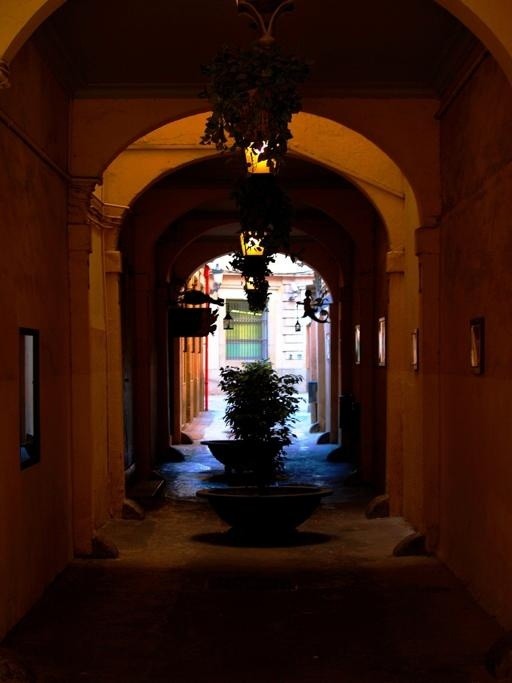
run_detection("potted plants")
[194,50,306,160]
[196,357,333,539]
[200,362,286,471]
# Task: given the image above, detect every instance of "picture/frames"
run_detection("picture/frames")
[376,315,386,366]
[467,315,486,373]
[409,327,419,372]
[13,323,41,469]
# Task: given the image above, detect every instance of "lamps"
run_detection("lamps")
[223,301,235,330]
[222,0,287,176]
[295,300,329,332]
